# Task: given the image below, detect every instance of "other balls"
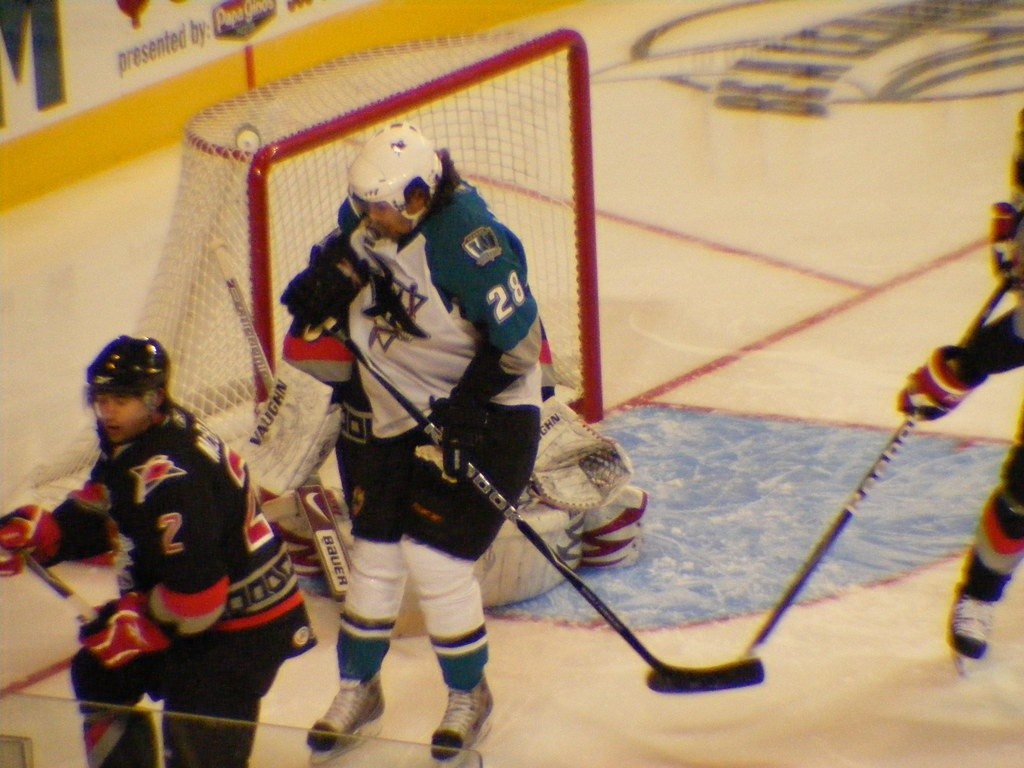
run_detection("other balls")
[235,123,263,152]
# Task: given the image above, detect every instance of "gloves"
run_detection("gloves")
[897,345,986,422]
[280,244,362,343]
[78,590,171,668]
[1,505,61,578]
[991,201,1024,288]
[415,397,492,487]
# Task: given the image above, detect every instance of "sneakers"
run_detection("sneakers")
[949,583,994,675]
[308,668,385,764]
[431,675,494,767]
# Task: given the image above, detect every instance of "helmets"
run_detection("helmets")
[86,334,170,395]
[347,121,444,216]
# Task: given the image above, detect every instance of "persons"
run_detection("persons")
[240,121,649,762]
[0,332,317,768]
[897,107,1024,657]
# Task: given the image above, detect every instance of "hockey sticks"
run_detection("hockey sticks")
[644,226,1024,695]
[210,229,431,644]
[0,511,101,628]
[321,316,767,700]
